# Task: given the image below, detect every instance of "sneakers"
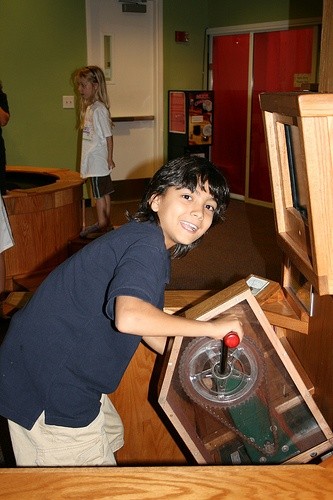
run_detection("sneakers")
[79,223,114,239]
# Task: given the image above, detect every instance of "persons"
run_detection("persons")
[0,156,244,465]
[0,89,10,166]
[71,65,116,236]
